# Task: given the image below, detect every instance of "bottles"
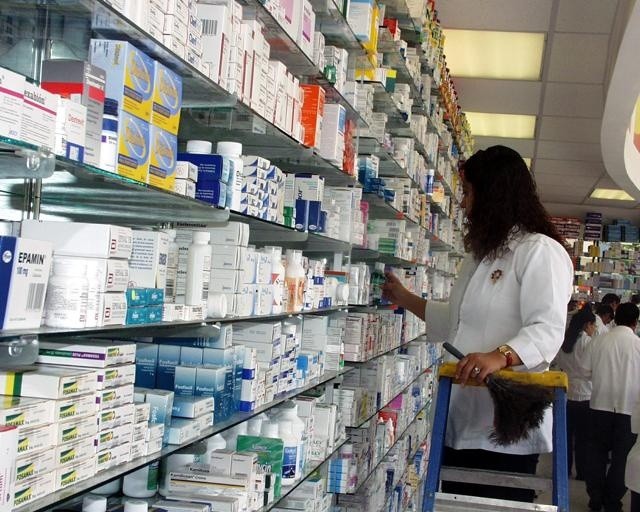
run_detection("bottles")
[200,403,304,486]
[82,460,159,512]
[98,96,117,173]
[377,417,393,446]
[161,228,227,319]
[340,446,356,494]
[426,168,435,195]
[248,243,314,314]
[370,262,386,306]
[186,140,242,211]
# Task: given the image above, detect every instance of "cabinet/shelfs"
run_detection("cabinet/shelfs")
[561,237,640,302]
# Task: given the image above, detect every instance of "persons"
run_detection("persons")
[560,292,640,511]
[377,144,574,502]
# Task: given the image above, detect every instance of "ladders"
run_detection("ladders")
[421,360,570,512]
